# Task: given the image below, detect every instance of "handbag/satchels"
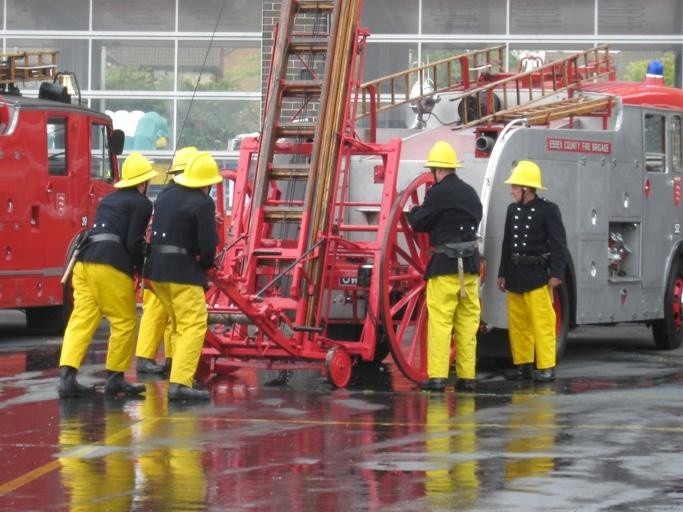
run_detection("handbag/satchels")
[444,240,476,260]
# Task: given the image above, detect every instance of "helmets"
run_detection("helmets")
[503,159,549,191]
[165,144,224,190]
[112,150,160,190]
[422,139,465,170]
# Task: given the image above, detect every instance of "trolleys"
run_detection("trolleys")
[138,0,485,397]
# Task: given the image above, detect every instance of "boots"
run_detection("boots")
[167,381,210,401]
[419,377,447,393]
[535,367,558,383]
[56,366,95,399]
[454,377,479,393]
[502,363,534,382]
[105,370,146,397]
[135,356,164,374]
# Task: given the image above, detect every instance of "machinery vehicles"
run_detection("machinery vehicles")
[0,41,128,335]
[224,66,682,349]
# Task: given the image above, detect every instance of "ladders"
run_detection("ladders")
[341,43,507,121]
[450,43,616,130]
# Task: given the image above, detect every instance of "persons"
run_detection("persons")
[496,160,570,383]
[134,145,199,373]
[56,150,157,398]
[407,140,483,391]
[151,150,224,400]
[457,73,501,124]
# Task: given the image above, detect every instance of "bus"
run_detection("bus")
[44,147,257,213]
